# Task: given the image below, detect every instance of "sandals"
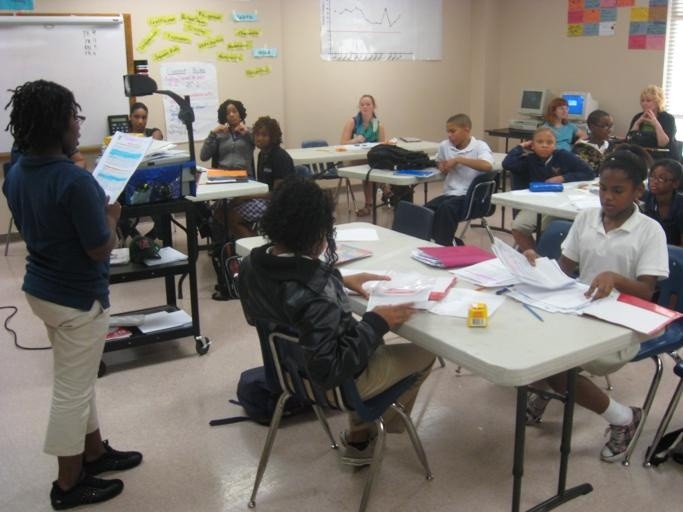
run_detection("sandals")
[355,204,372,218]
[380,183,394,198]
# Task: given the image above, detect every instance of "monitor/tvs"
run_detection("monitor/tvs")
[517,88,554,120]
[559,90,599,127]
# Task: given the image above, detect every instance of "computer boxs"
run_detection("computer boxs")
[508,120,544,131]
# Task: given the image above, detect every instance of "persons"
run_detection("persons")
[113,102,163,242]
[212,114,294,302]
[523,151,671,463]
[500,85,683,252]
[2,80,143,511]
[422,112,495,247]
[340,94,394,218]
[237,173,436,469]
[195,99,255,257]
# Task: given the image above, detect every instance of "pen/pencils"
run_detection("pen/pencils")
[496,284,514,295]
[522,302,544,322]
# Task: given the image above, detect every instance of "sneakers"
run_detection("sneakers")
[82,439,142,477]
[599,404,646,463]
[50,475,123,510]
[339,427,376,467]
[525,388,554,426]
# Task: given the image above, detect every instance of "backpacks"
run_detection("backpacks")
[207,239,245,301]
[382,184,415,208]
[236,365,317,426]
[366,143,437,170]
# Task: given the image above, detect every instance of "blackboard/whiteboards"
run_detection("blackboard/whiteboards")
[0,10,136,160]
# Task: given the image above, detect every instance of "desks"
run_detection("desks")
[485,127,534,152]
[179,167,269,298]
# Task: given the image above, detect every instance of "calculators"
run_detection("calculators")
[108,115,129,134]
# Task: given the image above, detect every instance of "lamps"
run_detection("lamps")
[123,73,196,174]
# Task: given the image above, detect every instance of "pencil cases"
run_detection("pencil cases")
[529,182,564,191]
